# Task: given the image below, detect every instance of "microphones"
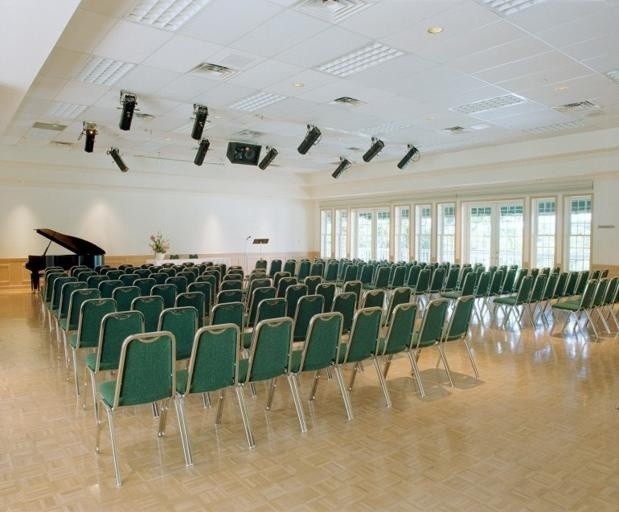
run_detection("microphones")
[246,236,251,240]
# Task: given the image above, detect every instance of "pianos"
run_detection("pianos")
[25,229,105,291]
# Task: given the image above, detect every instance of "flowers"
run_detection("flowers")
[148,232,170,252]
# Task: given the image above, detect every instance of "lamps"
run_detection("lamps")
[76,120,97,153]
[396,144,419,170]
[331,158,349,179]
[297,126,321,155]
[106,146,128,174]
[362,139,384,163]
[113,90,139,131]
[258,146,279,169]
[190,105,209,139]
[191,139,211,166]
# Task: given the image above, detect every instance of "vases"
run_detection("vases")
[156,253,164,259]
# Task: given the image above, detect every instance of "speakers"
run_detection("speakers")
[225,141,262,166]
